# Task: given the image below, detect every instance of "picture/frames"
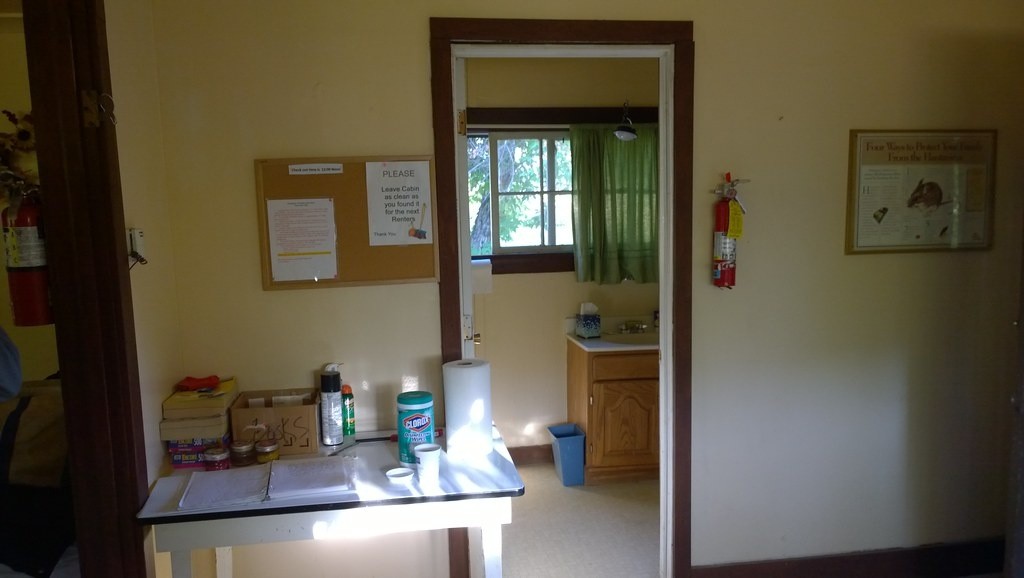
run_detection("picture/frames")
[253,154,440,291]
[844,129,999,253]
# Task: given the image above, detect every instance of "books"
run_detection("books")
[160,376,239,438]
[177,455,353,511]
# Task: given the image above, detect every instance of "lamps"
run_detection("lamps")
[613,99,637,141]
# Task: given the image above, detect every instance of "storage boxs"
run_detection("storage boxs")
[161,377,240,419]
[230,388,320,456]
[160,413,228,441]
[231,385,322,459]
[167,430,230,453]
[169,447,231,470]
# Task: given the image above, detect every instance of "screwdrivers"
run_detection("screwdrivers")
[355,427,444,444]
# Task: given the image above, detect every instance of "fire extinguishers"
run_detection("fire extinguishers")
[0,171,53,328]
[709,171,753,288]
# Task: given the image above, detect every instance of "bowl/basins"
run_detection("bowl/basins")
[385,468,415,482]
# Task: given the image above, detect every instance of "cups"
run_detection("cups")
[413,443,441,484]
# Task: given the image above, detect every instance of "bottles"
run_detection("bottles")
[320,370,356,451]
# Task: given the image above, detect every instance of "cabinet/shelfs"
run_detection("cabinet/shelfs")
[566,332,660,485]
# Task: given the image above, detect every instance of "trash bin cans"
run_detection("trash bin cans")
[546,421,586,487]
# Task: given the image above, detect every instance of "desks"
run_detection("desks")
[135,425,526,578]
